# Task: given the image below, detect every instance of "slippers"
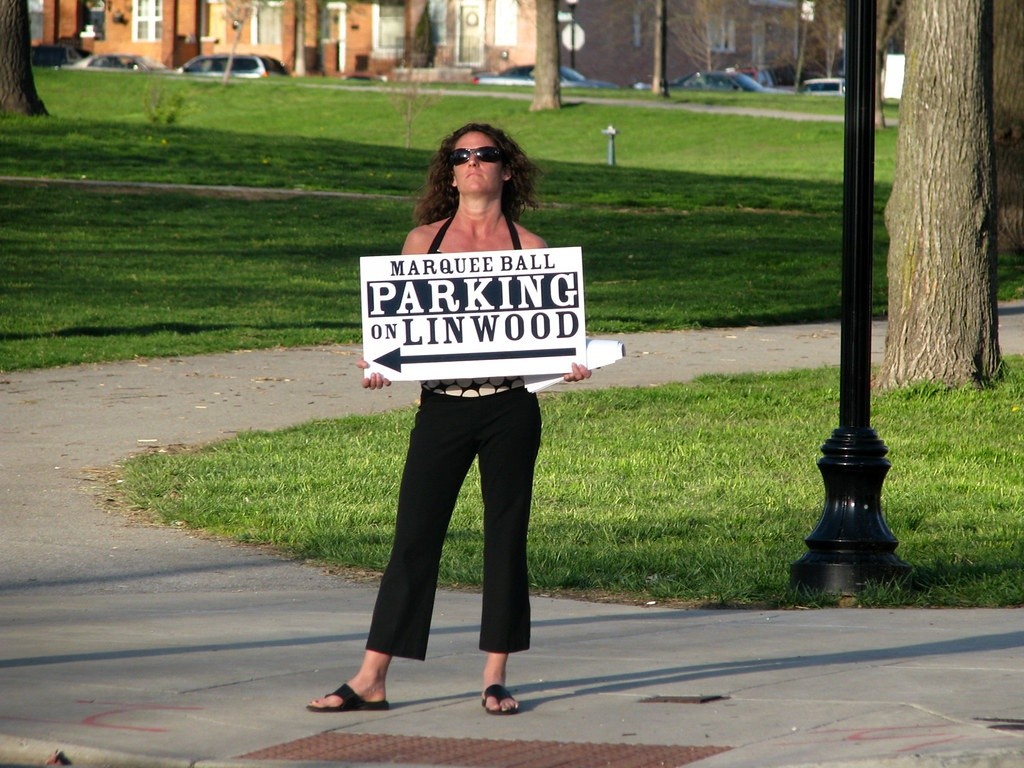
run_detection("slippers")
[306,682,390,712]
[481,684,520,716]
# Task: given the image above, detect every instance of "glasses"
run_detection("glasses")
[447,146,502,166]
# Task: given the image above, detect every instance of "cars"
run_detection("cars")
[31,42,91,69]
[473,64,620,91]
[800,77,847,95]
[633,68,796,97]
[59,52,173,73]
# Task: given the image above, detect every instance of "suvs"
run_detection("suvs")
[164,53,292,81]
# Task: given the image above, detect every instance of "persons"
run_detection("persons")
[306,124,623,715]
